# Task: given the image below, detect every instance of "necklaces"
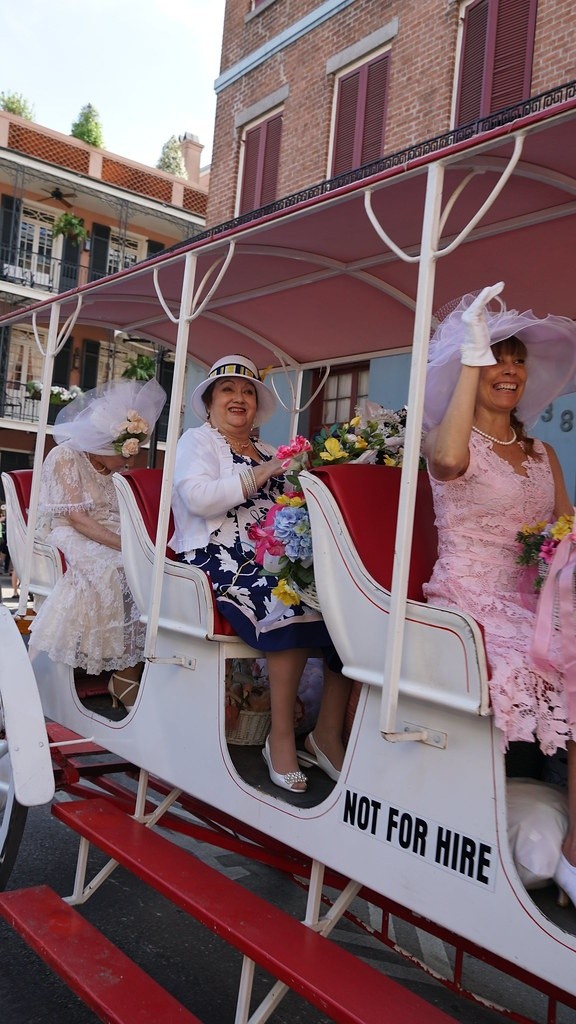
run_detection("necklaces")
[473,426,517,445]
[226,439,279,494]
[87,452,106,472]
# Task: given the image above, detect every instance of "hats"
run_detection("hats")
[190,354,277,430]
[422,289,576,430]
[51,375,167,458]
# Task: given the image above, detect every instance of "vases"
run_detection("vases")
[46,405,66,425]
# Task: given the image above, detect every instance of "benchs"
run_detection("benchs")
[0,465,576,1010]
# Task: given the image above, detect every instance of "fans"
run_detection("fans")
[37,187,78,208]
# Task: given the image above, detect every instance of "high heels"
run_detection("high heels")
[108,673,140,713]
[553,850,576,907]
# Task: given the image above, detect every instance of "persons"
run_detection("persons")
[12,570,34,601]
[421,281,576,906]
[0,509,10,575]
[26,378,167,712]
[167,355,350,794]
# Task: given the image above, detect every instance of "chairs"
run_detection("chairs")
[30,270,53,292]
[3,263,29,286]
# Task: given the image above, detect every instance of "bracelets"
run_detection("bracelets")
[239,468,257,497]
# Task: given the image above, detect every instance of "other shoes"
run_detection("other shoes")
[28,596,33,601]
[12,595,19,598]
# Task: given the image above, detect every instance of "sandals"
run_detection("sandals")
[296,731,341,781]
[262,734,307,792]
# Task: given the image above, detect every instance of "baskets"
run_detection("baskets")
[226,710,302,745]
[538,558,576,633]
[291,580,321,612]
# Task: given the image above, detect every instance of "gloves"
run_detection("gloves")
[460,282,505,367]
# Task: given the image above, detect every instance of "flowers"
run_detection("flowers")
[244,401,409,610]
[513,514,575,590]
[23,379,84,406]
[111,410,150,460]
[224,659,304,729]
